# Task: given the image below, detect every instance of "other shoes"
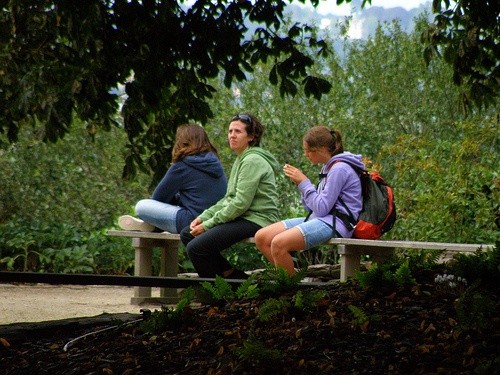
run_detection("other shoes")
[118,215,154,232]
[227,271,249,291]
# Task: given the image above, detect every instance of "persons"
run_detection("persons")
[118,123,228,233]
[179,113,280,292]
[254,125,364,279]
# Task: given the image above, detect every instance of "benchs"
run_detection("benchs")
[105,229,497,305]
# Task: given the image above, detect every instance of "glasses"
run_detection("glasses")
[232,114,251,124]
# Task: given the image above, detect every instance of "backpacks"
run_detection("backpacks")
[319,160,396,239]
